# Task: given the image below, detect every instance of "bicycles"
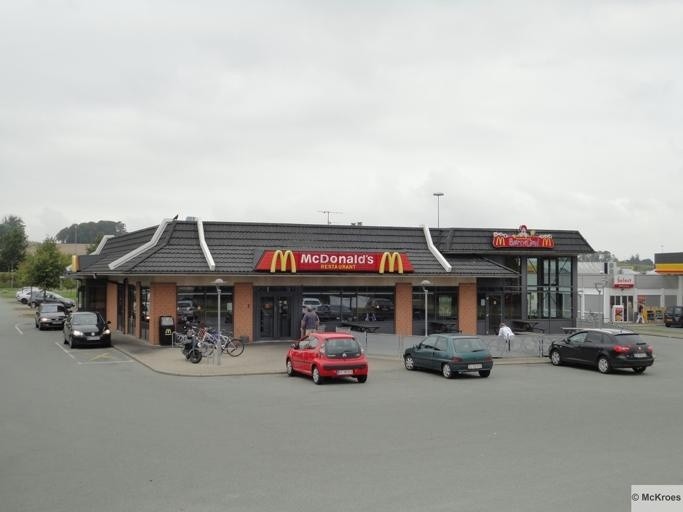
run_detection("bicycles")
[176,323,244,363]
[177,306,200,331]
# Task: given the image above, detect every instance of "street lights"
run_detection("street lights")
[432,192,444,228]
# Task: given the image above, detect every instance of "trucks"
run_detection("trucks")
[663,305,682,327]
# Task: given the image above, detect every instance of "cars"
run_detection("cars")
[62,311,111,348]
[34,303,73,330]
[277,297,497,322]
[15,286,75,308]
[402,333,493,378]
[547,328,655,374]
[131,289,150,328]
[285,332,368,384]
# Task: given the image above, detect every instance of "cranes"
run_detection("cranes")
[316,210,344,224]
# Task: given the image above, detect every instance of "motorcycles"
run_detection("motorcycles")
[224,311,231,323]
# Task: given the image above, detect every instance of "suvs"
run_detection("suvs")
[177,298,198,320]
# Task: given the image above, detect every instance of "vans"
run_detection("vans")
[177,300,197,318]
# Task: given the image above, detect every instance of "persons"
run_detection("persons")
[498,323,515,340]
[518,225,529,237]
[300,305,320,338]
